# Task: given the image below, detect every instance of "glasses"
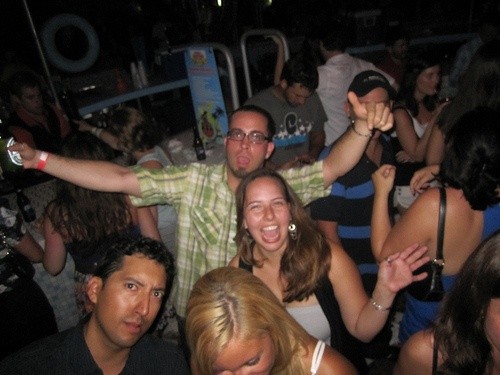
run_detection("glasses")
[226,130,270,144]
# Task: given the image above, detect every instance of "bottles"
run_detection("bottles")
[0,117,25,176]
[16,187,36,223]
[193,127,206,161]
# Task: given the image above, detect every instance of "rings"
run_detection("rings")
[385,256,393,263]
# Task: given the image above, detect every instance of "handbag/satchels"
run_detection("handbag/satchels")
[401,187,448,302]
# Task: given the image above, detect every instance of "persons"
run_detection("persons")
[0,22,500,375]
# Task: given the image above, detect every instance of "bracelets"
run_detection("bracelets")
[351,120,372,138]
[91,126,99,135]
[36,151,50,170]
[369,299,393,312]
[96,128,103,139]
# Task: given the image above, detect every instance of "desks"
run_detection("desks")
[2,128,226,332]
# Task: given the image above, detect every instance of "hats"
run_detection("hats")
[347,69,398,101]
